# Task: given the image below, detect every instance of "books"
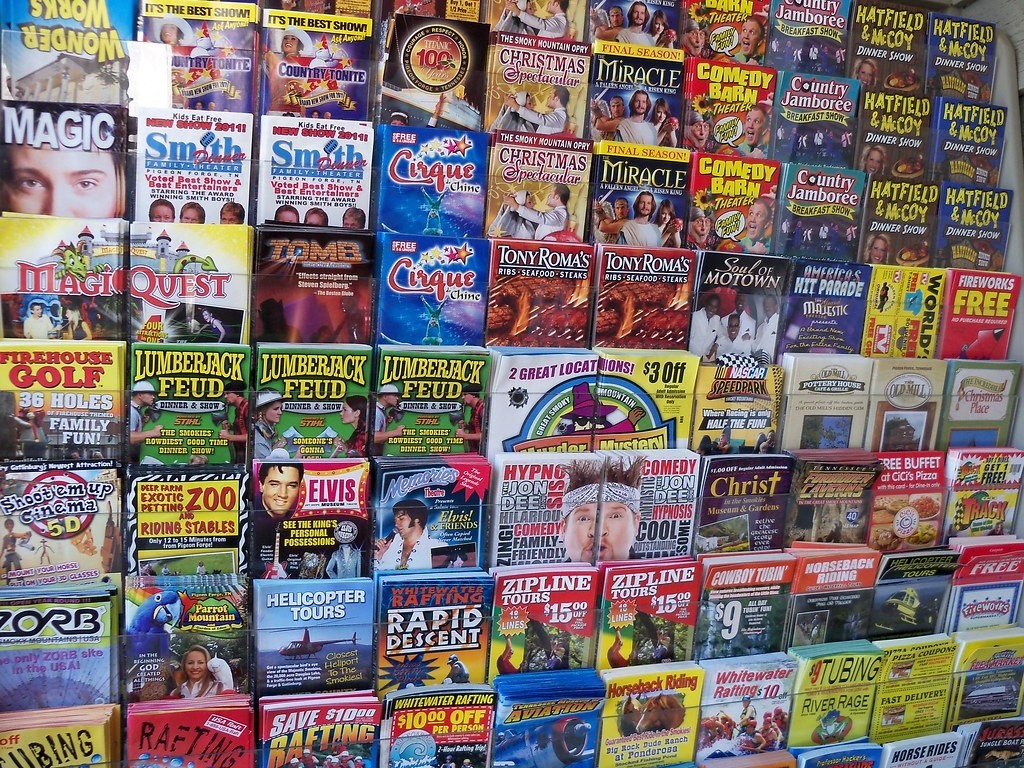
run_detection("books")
[0,1,1023,766]
